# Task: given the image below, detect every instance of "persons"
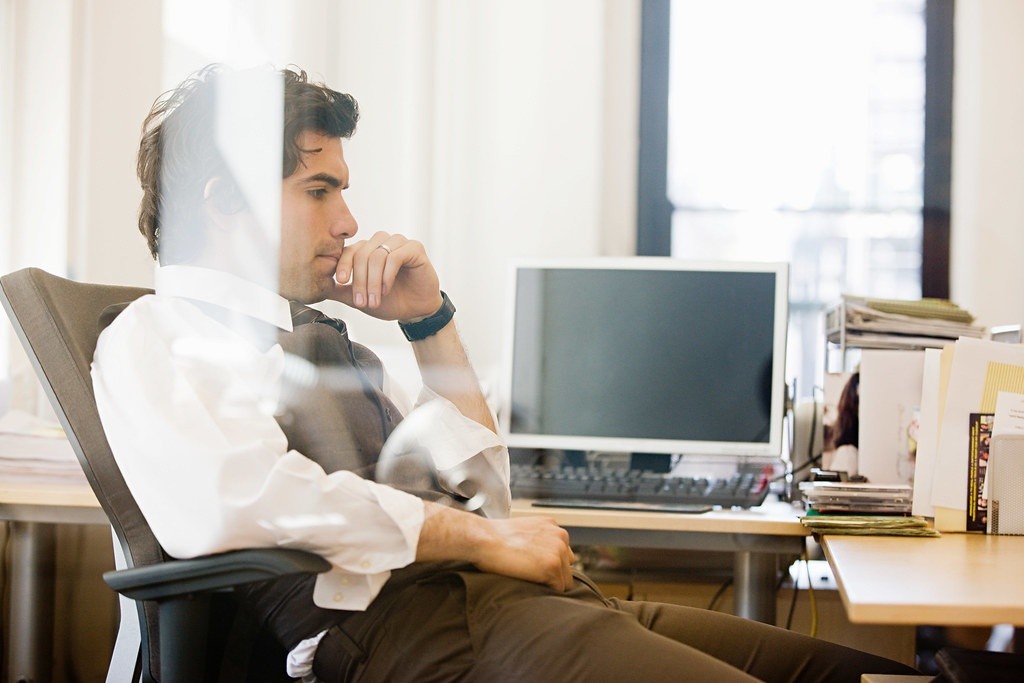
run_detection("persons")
[89,62,945,683]
[826,372,860,479]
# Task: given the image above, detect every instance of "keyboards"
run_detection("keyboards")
[508,463,770,506]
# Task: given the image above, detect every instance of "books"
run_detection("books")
[799,294,1024,535]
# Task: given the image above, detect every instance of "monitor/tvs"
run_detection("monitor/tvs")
[498,259,789,477]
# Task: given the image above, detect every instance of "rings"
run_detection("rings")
[378,244,393,255]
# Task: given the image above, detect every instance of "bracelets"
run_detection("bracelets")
[398,291,457,342]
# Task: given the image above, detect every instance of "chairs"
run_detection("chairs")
[0,268,334,683]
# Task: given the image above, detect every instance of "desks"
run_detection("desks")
[0,478,1024,683]
